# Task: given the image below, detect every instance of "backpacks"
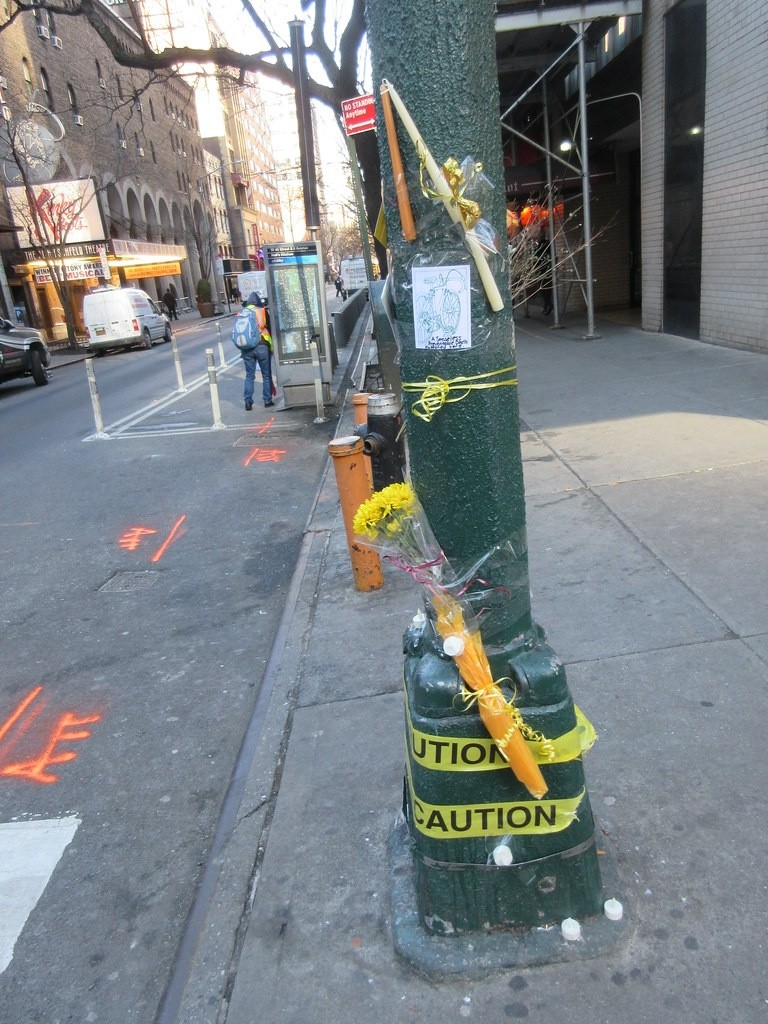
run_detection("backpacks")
[231,306,263,351]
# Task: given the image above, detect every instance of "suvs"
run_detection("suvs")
[1,317,54,385]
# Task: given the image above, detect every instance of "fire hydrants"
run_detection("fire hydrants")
[356,386,408,490]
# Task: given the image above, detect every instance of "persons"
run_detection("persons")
[162,289,179,321]
[232,292,274,411]
[335,277,342,296]
[538,240,554,316]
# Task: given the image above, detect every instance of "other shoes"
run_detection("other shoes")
[175,319,178,320]
[171,319,172,321]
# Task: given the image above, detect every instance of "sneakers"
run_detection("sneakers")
[245,401,252,410]
[265,401,275,407]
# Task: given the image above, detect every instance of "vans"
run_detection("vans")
[84,282,173,353]
[236,269,268,309]
[340,256,379,301]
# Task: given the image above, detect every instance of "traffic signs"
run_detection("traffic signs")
[338,94,380,138]
